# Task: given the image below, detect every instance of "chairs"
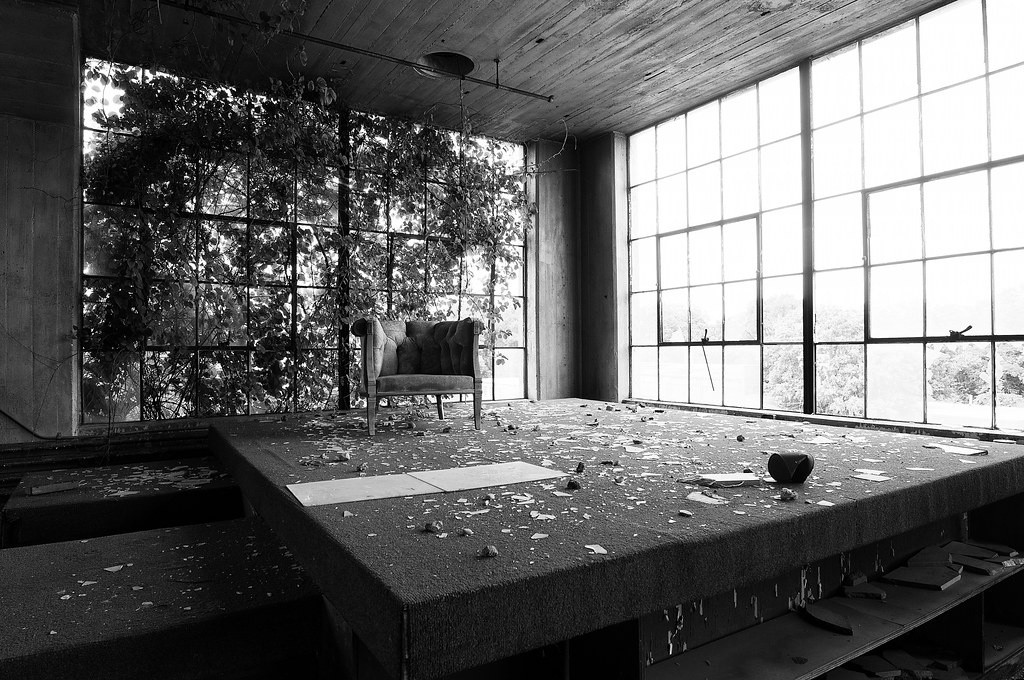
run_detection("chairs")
[351,319,488,436]
